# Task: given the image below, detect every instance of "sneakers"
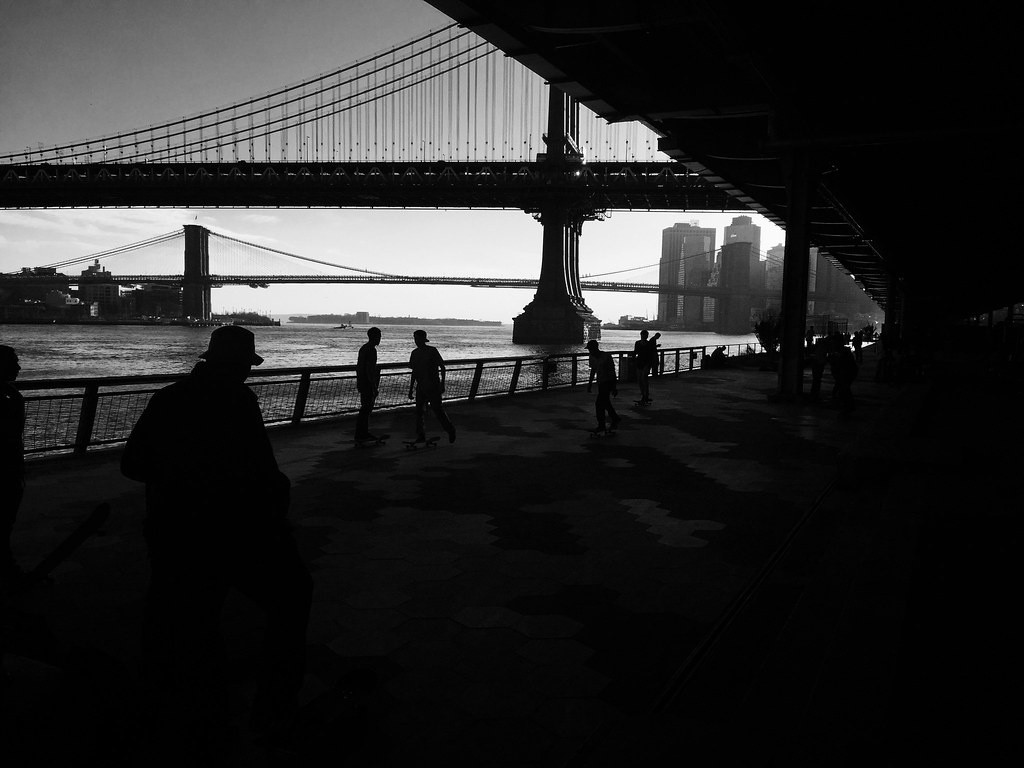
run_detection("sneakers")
[587,423,606,432]
[449,429,455,443]
[416,437,425,443]
[358,435,378,441]
[608,418,622,430]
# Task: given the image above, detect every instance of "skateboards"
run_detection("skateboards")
[350,434,390,447]
[402,436,441,450]
[586,426,619,440]
[634,399,653,407]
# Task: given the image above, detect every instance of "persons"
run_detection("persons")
[0,345,30,587]
[631,330,654,403]
[584,340,621,432]
[712,346,726,367]
[810,331,862,396]
[408,330,456,443]
[122,326,291,768]
[354,327,381,440]
[650,333,661,377]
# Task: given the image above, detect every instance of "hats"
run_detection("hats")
[199,326,264,366]
[585,340,598,348]
[413,329,429,342]
